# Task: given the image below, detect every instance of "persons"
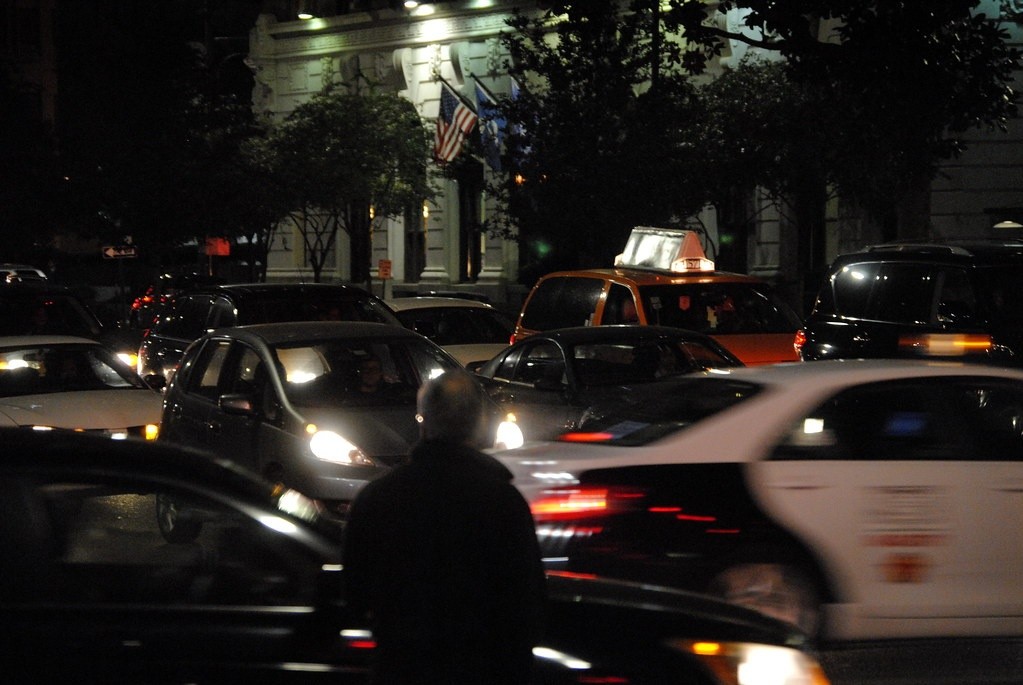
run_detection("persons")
[341,371,553,683]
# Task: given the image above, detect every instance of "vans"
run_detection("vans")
[795,238,1023,378]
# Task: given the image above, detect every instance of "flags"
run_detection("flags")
[433,82,477,168]
[475,76,543,177]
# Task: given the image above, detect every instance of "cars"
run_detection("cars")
[469,358,1023,646]
[377,297,512,377]
[0,265,185,335]
[157,318,490,563]
[0,336,161,499]
[463,323,749,386]
[510,268,806,370]
[137,279,405,399]
[0,281,140,377]
